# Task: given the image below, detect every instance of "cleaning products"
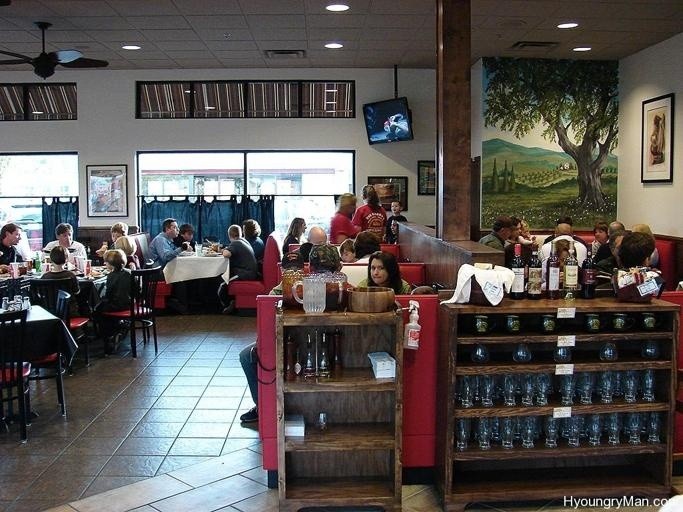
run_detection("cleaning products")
[402,301,423,352]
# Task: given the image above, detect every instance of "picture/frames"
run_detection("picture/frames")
[86,164,128,217]
[367,176,408,212]
[641,93,674,183]
[417,160,435,196]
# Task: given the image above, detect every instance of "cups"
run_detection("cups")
[521,415,537,449]
[587,415,603,447]
[502,375,517,407]
[641,370,656,404]
[598,372,616,405]
[535,373,549,407]
[311,410,329,433]
[325,274,349,313]
[460,376,474,408]
[579,373,596,406]
[42,262,52,274]
[606,413,625,447]
[579,416,589,439]
[18,261,29,276]
[542,415,561,449]
[567,415,585,448]
[559,417,570,441]
[195,244,202,258]
[291,276,327,314]
[455,419,471,451]
[646,412,661,444]
[9,262,20,280]
[511,418,522,440]
[476,418,493,451]
[560,374,577,406]
[490,419,501,441]
[73,256,78,271]
[498,417,515,449]
[78,257,84,272]
[479,375,494,408]
[622,371,639,403]
[83,260,92,277]
[627,412,644,447]
[520,373,535,407]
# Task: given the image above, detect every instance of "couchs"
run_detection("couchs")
[660,290,683,476]
[530,235,675,291]
[115,232,172,316]
[257,295,439,488]
[277,262,426,287]
[288,244,400,258]
[228,231,282,316]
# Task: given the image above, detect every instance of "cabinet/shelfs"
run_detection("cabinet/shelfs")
[274,301,402,512]
[435,288,681,512]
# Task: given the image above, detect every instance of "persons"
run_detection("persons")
[218,219,265,280]
[173,224,196,252]
[357,251,412,295]
[597,230,628,275]
[96,222,128,266]
[504,216,521,248]
[354,231,381,263]
[38,246,80,319]
[291,227,327,254]
[386,201,408,244]
[391,217,404,246]
[218,225,258,314]
[309,245,348,291]
[300,243,313,263]
[92,249,137,352]
[596,232,667,292]
[283,218,307,256]
[115,236,143,304]
[478,216,513,252]
[543,216,588,260]
[592,224,609,262]
[538,223,587,289]
[330,194,362,244]
[240,253,306,422]
[41,223,87,265]
[505,243,532,268]
[592,222,625,264]
[632,224,658,268]
[144,220,193,311]
[0,223,25,274]
[351,186,387,242]
[515,216,537,245]
[340,240,361,263]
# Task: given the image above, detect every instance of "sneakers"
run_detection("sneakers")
[240,407,258,422]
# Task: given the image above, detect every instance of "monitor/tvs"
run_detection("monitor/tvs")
[363,97,414,145]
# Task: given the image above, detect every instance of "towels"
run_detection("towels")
[440,264,515,306]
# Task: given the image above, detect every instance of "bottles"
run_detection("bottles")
[611,313,626,332]
[304,332,316,378]
[474,316,488,335]
[504,316,520,336]
[562,241,578,299]
[641,312,656,330]
[510,244,525,299]
[585,314,600,333]
[318,331,330,377]
[547,241,560,299]
[284,336,295,380]
[333,329,340,370]
[540,315,555,335]
[36,253,41,272]
[626,314,637,331]
[656,314,664,330]
[582,243,593,299]
[527,243,542,299]
[294,347,303,375]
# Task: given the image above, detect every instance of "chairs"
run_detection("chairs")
[29,277,89,375]
[0,310,31,440]
[89,254,104,266]
[29,289,71,417]
[99,265,162,358]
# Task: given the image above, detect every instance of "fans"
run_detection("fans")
[0,50,109,80]
